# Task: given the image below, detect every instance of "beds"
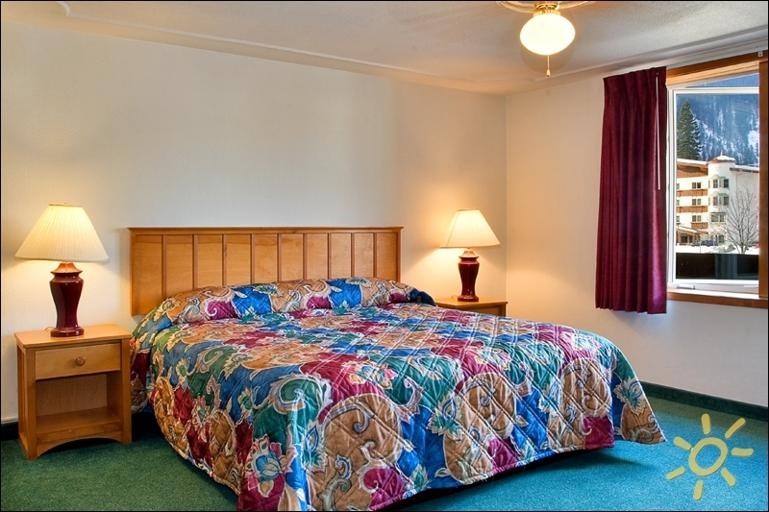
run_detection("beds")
[118,227,616,510]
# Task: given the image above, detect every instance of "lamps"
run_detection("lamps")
[495,0,595,77]
[435,208,501,301]
[15,203,109,337]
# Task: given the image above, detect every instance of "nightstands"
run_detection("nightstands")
[14,324,133,459]
